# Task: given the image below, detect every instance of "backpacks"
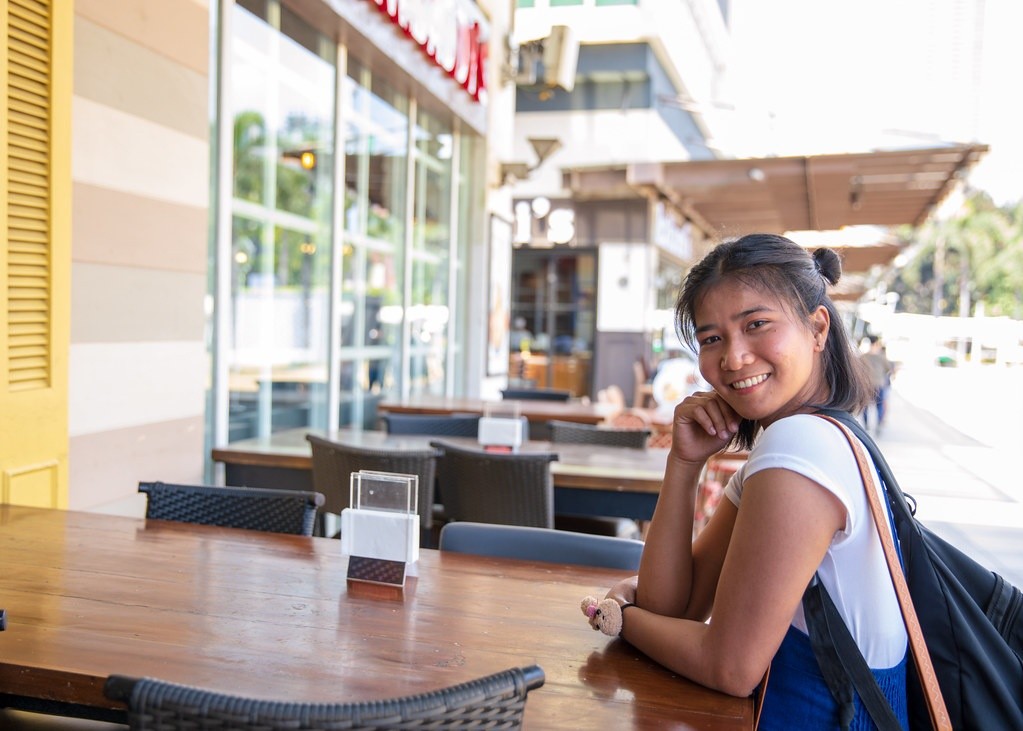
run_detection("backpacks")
[816,409,1023,730]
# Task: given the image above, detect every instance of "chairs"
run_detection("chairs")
[429,439,562,532]
[137,479,327,538]
[101,663,546,728]
[437,522,648,574]
[380,408,483,445]
[304,433,445,530]
[548,419,657,446]
[500,387,572,401]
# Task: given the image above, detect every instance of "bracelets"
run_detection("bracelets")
[579,596,636,635]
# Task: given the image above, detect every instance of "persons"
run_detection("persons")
[848,287,892,438]
[580,234,919,731]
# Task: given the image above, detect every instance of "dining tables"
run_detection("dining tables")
[377,397,615,426]
[210,429,674,527]
[0,502,756,731]
